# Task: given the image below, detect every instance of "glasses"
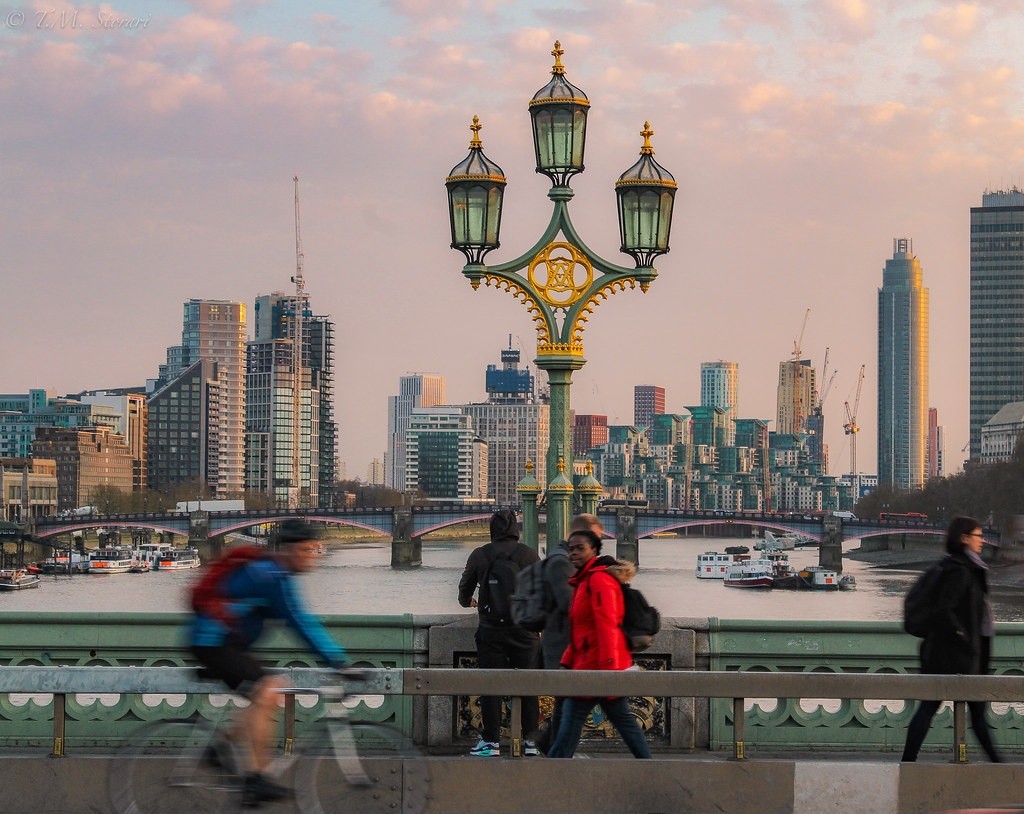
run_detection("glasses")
[966,533,984,539]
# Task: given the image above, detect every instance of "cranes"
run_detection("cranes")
[791,308,838,445]
[292,176,306,484]
[844,364,867,508]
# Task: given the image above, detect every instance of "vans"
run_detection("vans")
[833,512,859,523]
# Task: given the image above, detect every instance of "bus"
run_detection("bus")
[879,511,928,526]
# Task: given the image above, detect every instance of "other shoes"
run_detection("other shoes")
[240,774,299,808]
[202,743,242,785]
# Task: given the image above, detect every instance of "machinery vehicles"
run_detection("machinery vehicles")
[62,506,98,516]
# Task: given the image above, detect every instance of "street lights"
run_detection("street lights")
[444,42,678,561]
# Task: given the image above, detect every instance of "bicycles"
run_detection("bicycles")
[106,660,430,814]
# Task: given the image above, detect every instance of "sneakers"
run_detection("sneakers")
[469,740,500,757]
[524,739,539,755]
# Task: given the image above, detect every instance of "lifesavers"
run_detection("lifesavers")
[751,567,756,572]
[767,568,771,571]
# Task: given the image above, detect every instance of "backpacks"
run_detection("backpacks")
[510,549,570,632]
[481,542,527,629]
[190,545,279,628]
[903,552,960,637]
[612,577,662,654]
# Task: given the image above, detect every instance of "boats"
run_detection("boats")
[723,560,774,588]
[86,545,133,574]
[839,573,858,589]
[157,546,201,569]
[0,568,39,590]
[131,561,149,572]
[135,544,175,570]
[696,547,788,578]
[759,547,839,589]
[753,537,795,551]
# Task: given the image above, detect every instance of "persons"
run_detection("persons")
[901,511,1006,763]
[536,509,605,757]
[548,528,657,760]
[184,519,354,806]
[459,509,542,758]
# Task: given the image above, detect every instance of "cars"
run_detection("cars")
[804,515,817,521]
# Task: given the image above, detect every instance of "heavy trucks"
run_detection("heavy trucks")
[176,500,244,511]
